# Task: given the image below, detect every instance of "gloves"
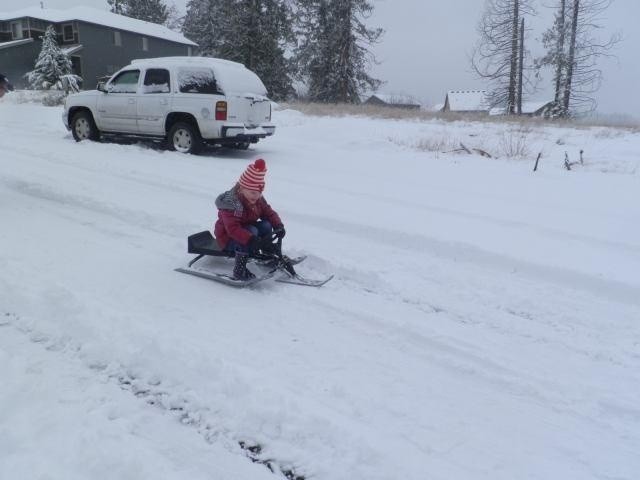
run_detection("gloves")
[274,225,287,239]
[246,233,265,251]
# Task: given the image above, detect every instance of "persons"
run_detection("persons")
[0,74,14,99]
[214,159,285,279]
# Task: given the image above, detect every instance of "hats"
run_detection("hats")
[237,158,269,193]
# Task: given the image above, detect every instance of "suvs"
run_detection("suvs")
[61,56,276,155]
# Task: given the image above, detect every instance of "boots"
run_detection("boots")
[231,249,257,282]
[258,231,281,255]
